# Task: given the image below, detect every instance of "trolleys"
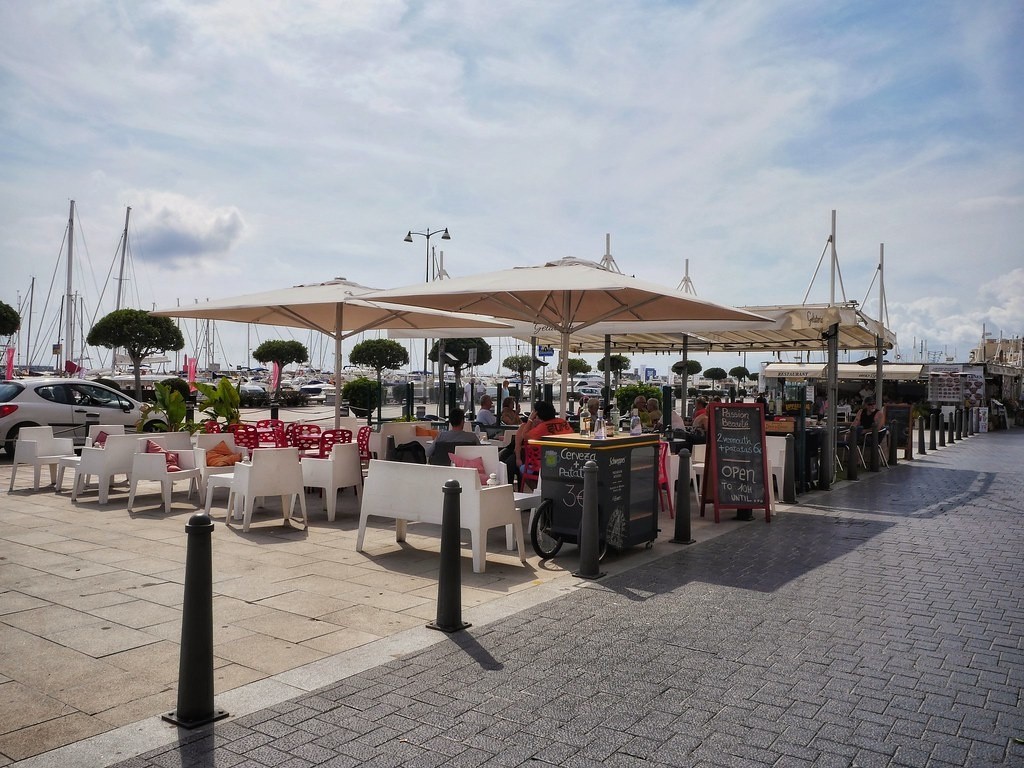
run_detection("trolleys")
[528,432,661,559]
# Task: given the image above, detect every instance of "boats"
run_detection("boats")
[552,372,605,393]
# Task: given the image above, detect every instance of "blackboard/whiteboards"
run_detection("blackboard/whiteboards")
[702,402,770,508]
[883,404,913,448]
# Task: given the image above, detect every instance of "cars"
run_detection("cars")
[0,378,170,457]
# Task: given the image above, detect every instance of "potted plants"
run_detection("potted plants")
[912,399,933,430]
[999,397,1018,425]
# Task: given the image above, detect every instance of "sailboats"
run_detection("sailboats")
[341,329,531,386]
[73,207,179,391]
[195,298,335,395]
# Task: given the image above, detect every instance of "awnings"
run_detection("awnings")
[837,363,924,381]
[764,363,828,379]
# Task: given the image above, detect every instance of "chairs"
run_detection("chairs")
[10,415,544,573]
[657,422,890,518]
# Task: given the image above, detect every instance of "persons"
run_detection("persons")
[424,381,886,493]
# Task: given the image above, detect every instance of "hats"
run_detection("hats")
[865,396,876,403]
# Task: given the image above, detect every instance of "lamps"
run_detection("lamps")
[856,349,888,366]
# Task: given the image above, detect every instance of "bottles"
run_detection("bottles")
[775,393,782,415]
[610,398,620,435]
[92,442,102,449]
[630,408,642,436]
[593,407,607,439]
[580,399,591,438]
[240,456,251,465]
[486,473,500,488]
[769,391,774,414]
[605,418,615,437]
[475,425,480,439]
[664,425,674,441]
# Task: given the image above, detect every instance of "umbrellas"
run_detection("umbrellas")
[145,275,515,431]
[354,250,778,420]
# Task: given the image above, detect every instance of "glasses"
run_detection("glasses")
[867,402,876,406]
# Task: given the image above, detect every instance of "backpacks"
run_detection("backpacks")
[394,441,427,464]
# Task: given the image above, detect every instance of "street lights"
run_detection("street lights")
[404,228,450,405]
